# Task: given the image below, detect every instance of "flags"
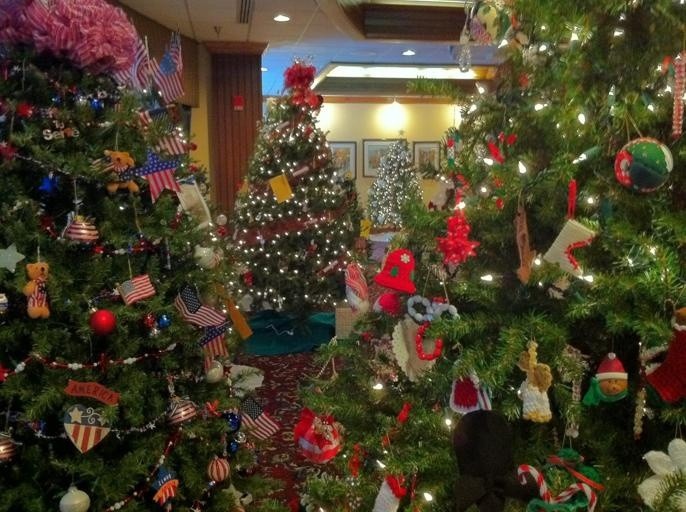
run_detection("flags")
[176,282,225,328]
[240,397,280,440]
[111,29,185,153]
[120,276,155,304]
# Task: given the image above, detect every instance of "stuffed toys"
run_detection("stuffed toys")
[582,352,630,407]
[26,263,50,317]
[109,151,139,192]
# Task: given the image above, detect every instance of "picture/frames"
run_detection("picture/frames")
[411,140,441,180]
[325,141,358,182]
[362,138,408,180]
